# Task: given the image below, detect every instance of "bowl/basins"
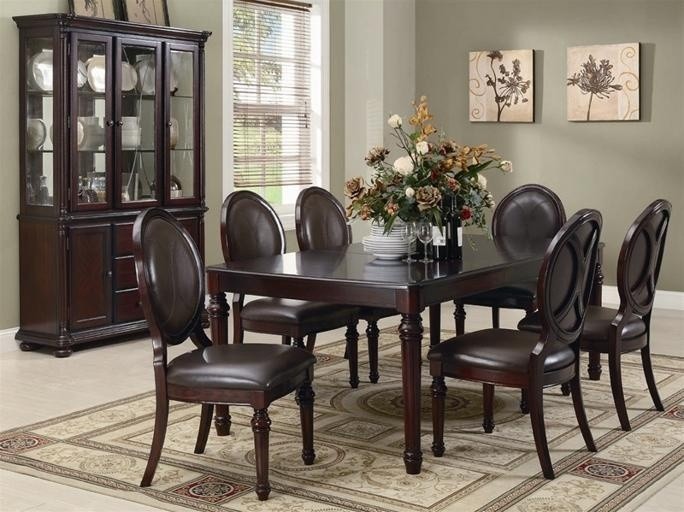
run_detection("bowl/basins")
[122,131,142,150]
[122,117,141,130]
[86,129,105,147]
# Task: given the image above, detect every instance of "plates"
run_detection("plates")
[369,224,405,239]
[136,60,177,95]
[121,60,138,92]
[26,48,53,91]
[77,58,88,87]
[87,55,104,93]
[361,236,408,259]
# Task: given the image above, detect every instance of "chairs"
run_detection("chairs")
[219,190,359,405]
[427,208,603,479]
[453,184,566,334]
[134,207,316,501]
[518,200,673,431]
[295,187,402,386]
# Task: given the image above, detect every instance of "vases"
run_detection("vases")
[411,217,464,260]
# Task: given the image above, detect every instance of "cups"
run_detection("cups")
[400,221,417,264]
[417,222,434,263]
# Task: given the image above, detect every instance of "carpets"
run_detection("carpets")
[1,322,683,512]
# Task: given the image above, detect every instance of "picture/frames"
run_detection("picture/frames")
[69,0,120,20]
[567,42,639,121]
[122,0,170,26]
[469,50,535,122]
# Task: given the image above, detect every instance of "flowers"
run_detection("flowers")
[344,95,513,252]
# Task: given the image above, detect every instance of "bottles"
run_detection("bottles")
[78,174,107,203]
[446,195,462,262]
[38,177,49,203]
[26,174,35,206]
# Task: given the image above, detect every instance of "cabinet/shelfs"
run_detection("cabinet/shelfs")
[12,13,213,358]
